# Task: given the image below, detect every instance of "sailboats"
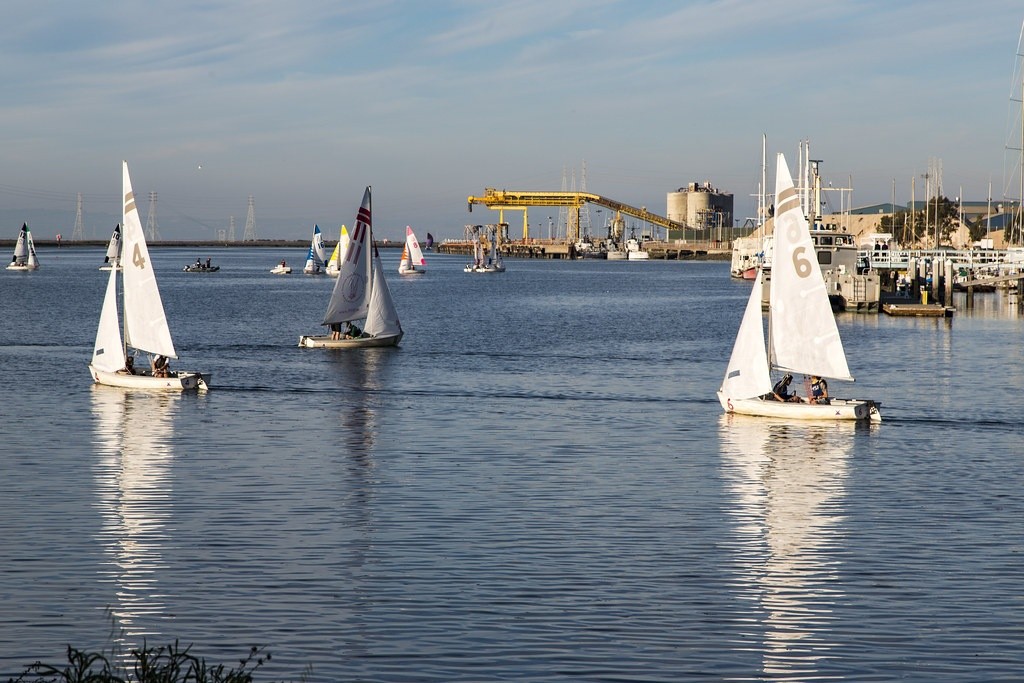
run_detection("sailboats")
[6,221,40,270]
[298,186,406,348]
[398,225,427,275]
[89,159,217,390]
[303,224,350,275]
[98,223,123,271]
[717,134,887,418]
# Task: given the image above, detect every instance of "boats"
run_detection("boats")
[463,267,506,272]
[182,265,220,272]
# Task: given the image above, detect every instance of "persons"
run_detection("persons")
[529,246,545,259]
[206,258,211,269]
[196,258,201,268]
[882,242,888,262]
[330,322,363,341]
[772,374,801,403]
[875,241,881,261]
[809,377,830,406]
[152,355,170,379]
